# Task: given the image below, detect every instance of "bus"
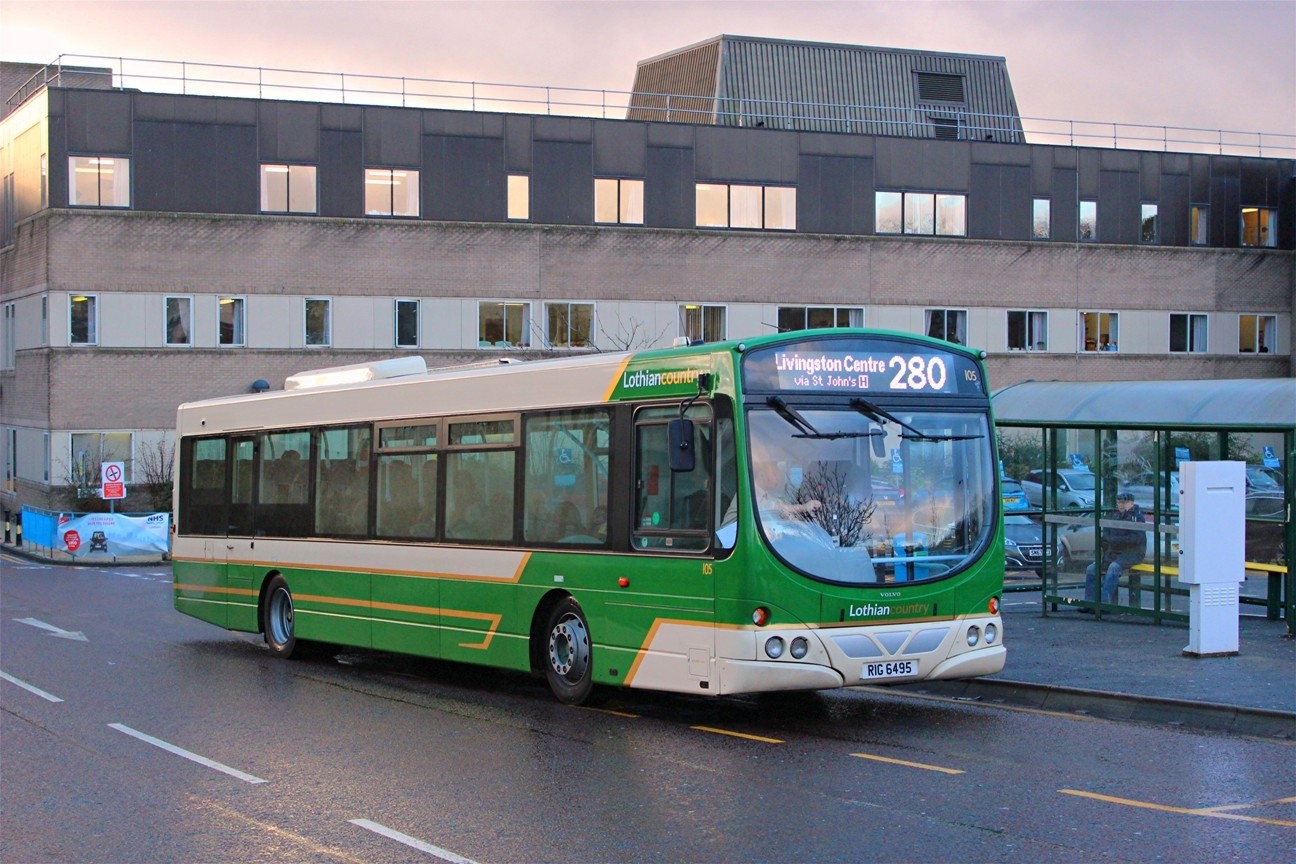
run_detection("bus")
[169,326,1009,706]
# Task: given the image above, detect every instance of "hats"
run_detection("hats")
[1116,493,1135,501]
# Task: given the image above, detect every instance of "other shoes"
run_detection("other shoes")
[1088,608,1109,615]
[1078,606,1090,613]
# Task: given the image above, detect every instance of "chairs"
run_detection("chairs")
[214,460,458,517]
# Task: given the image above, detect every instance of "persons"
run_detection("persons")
[1077,493,1147,613]
[272,450,603,549]
[721,464,822,540]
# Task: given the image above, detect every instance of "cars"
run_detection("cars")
[868,464,1284,579]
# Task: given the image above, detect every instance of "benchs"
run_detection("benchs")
[1131,559,1286,620]
